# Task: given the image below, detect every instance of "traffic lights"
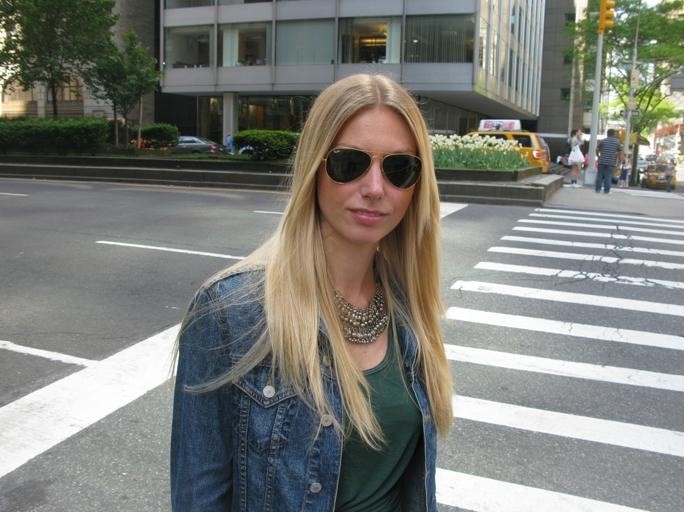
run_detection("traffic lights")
[604,0,616,28]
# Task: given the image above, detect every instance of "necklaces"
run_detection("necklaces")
[333,284,388,343]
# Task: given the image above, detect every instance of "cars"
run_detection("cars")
[146,135,216,153]
[641,162,677,190]
[239,142,298,156]
[635,153,679,173]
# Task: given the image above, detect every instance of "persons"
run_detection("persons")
[226,135,231,152]
[168,73,451,511]
[568,128,633,194]
[665,158,676,193]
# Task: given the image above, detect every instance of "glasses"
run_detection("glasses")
[323,148,422,190]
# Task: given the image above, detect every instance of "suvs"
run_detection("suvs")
[460,118,551,173]
